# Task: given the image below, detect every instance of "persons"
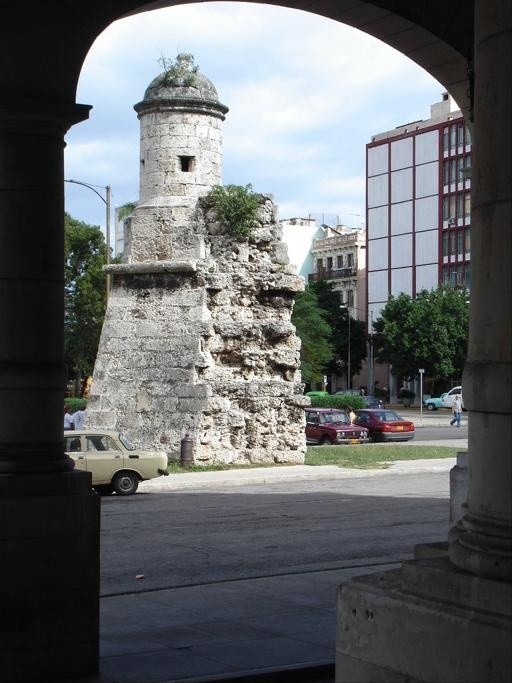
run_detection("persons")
[64,405,73,430]
[72,406,86,430]
[450,395,462,427]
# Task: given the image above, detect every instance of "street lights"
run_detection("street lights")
[339,304,351,389]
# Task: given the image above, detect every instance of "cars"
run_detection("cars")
[303,408,370,445]
[352,408,415,441]
[64,432,169,495]
[424,392,448,410]
[441,386,466,411]
